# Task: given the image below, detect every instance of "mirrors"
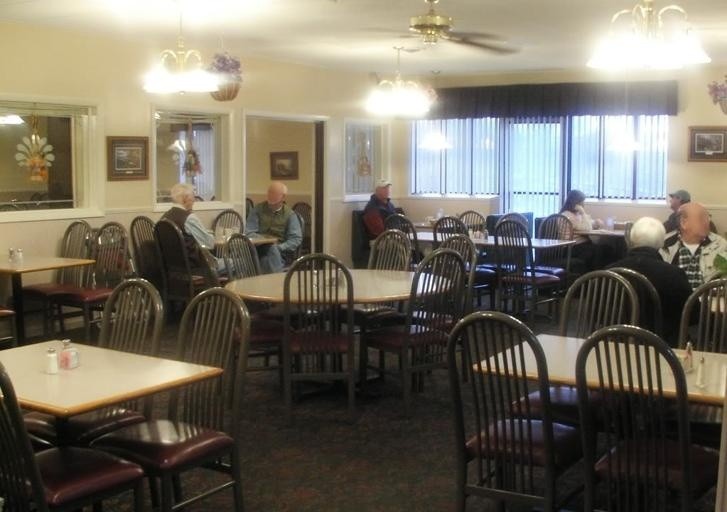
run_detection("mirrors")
[346,124,375,196]
[155,115,224,204]
[0,110,73,209]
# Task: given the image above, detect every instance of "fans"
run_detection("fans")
[355,0,516,58]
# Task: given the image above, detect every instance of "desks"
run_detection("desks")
[574,225,625,237]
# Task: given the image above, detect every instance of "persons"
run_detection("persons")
[364,180,399,239]
[665,190,691,232]
[660,202,727,298]
[161,183,242,278]
[559,190,594,239]
[602,217,700,347]
[246,182,304,272]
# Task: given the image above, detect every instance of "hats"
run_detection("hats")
[668,190,690,202]
[375,179,392,189]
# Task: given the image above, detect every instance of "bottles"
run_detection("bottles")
[207,229,215,237]
[484,229,490,241]
[337,268,347,288]
[467,229,474,239]
[45,348,59,374]
[58,339,79,369]
[8,247,24,262]
[695,357,709,389]
[686,341,695,368]
[313,269,319,288]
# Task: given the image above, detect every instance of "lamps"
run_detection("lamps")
[142,0,220,95]
[27,114,41,140]
[359,46,438,123]
[584,0,710,77]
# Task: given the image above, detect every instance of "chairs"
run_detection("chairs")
[284,250,357,418]
[505,270,641,438]
[398,234,479,386]
[444,306,623,512]
[92,282,250,511]
[2,362,147,512]
[21,275,165,454]
[645,278,726,451]
[577,321,721,512]
[352,203,574,318]
[1,206,285,350]
[340,228,410,373]
[365,247,468,416]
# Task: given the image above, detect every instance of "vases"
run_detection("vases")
[214,82,240,101]
[719,99,727,114]
[709,78,727,98]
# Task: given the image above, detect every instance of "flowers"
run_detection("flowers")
[211,53,242,81]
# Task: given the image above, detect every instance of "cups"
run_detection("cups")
[474,231,482,240]
[224,228,232,239]
[606,215,615,232]
[423,216,448,225]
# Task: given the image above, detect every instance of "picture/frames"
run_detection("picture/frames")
[270,151,299,180]
[105,135,148,182]
[688,124,726,163]
